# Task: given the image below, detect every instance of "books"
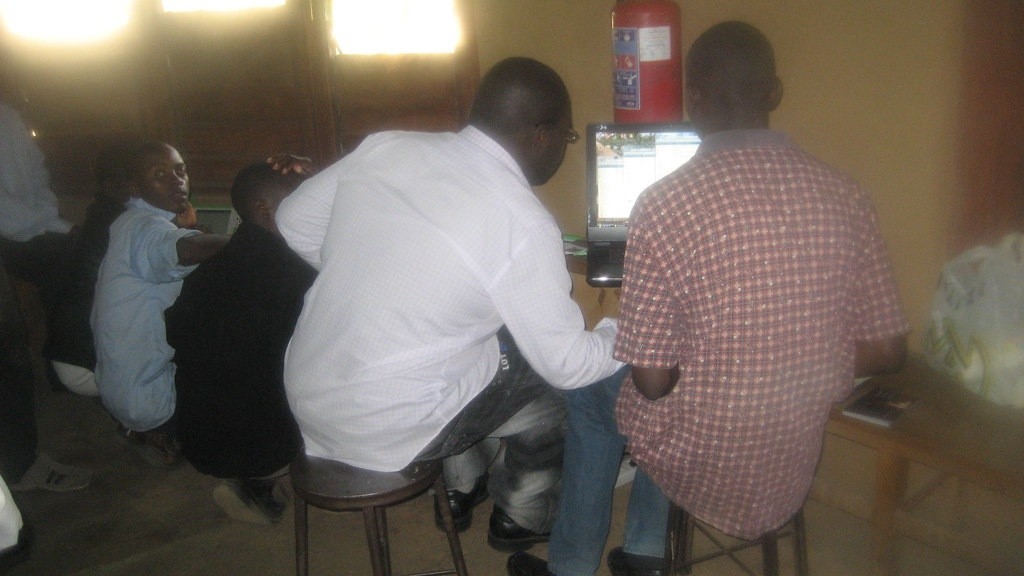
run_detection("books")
[842,386,922,428]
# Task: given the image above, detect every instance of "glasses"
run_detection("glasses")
[535,120,579,144]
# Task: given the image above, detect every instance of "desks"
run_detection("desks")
[808,370,1024,576]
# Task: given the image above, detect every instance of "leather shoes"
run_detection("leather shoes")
[506,551,557,576]
[488,503,552,553]
[434,470,490,535]
[606,547,664,576]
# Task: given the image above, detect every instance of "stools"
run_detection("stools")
[668,507,810,575]
[293,452,467,576]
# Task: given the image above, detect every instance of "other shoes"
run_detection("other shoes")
[116,423,185,464]
[210,479,290,527]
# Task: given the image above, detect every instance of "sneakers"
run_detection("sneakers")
[10,452,93,492]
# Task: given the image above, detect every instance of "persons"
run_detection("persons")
[93,142,237,470]
[43,145,137,402]
[274,58,630,552]
[0,62,82,491]
[506,23,914,575]
[164,152,321,525]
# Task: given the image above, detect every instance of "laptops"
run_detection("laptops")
[586,122,702,287]
[191,207,237,236]
